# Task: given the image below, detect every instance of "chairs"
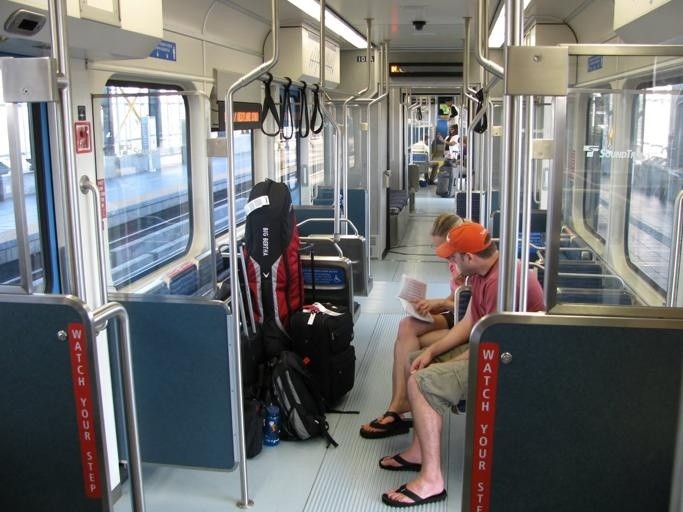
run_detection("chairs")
[516,224,630,303]
[409,151,429,185]
[294,185,371,325]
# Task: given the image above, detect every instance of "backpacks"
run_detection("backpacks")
[267,358,340,449]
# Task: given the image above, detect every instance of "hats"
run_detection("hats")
[434,220,491,259]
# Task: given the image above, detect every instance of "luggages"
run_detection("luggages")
[290,242,357,399]
[436,172,451,197]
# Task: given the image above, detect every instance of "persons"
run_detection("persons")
[435,124,460,167]
[444,97,459,136]
[378,220,543,508]
[412,136,439,186]
[359,212,472,441]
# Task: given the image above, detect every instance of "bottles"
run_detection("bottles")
[262,404,281,446]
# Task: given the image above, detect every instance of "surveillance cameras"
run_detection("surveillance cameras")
[413,21,426,31]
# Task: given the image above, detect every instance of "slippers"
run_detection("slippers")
[369,411,418,430]
[378,452,424,472]
[381,481,448,507]
[359,424,412,439]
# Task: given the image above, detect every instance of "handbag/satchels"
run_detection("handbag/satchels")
[243,178,306,323]
